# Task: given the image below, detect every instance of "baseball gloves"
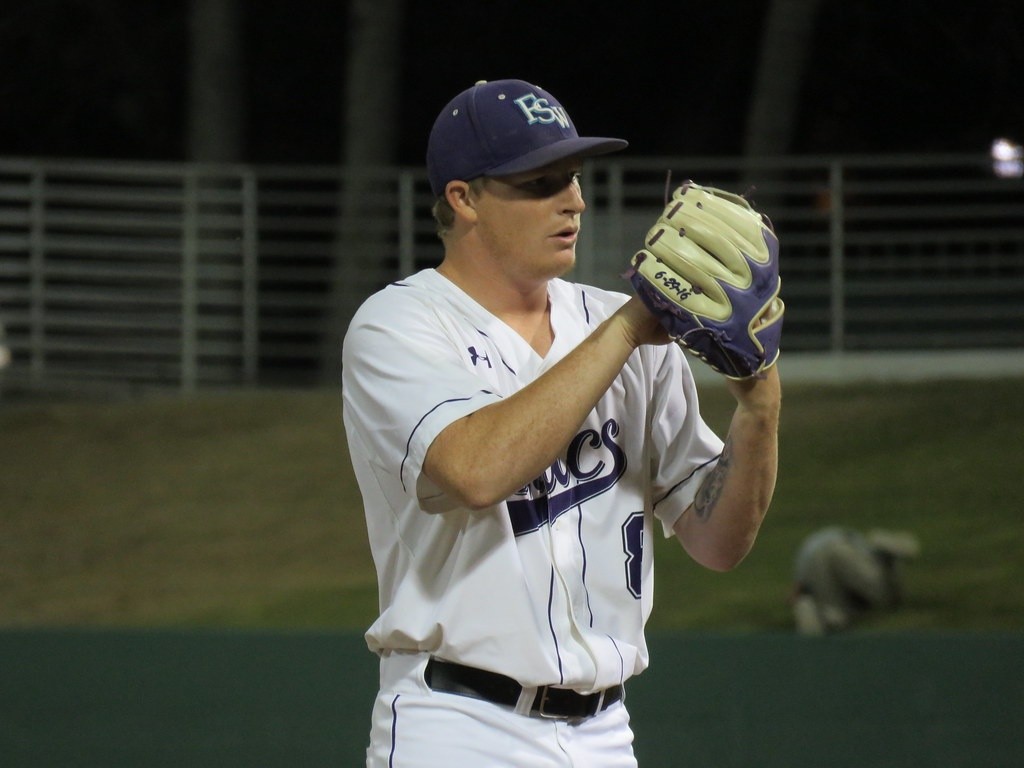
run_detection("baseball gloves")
[624,178,788,382]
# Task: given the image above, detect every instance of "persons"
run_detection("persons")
[341,77,783,768]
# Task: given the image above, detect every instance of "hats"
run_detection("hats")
[426,78,629,198]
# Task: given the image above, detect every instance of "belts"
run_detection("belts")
[424,656,623,720]
